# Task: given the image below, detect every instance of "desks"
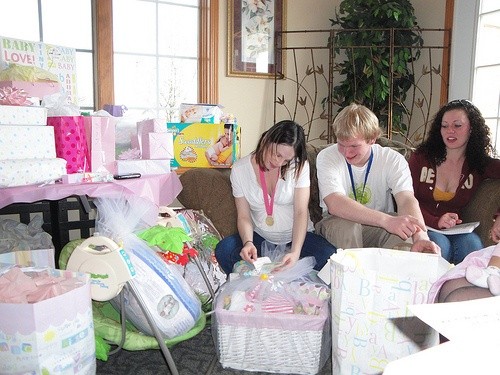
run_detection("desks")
[0,170,183,271]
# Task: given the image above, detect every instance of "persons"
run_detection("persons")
[214,120,337,277]
[313,102,442,255]
[205,124,233,167]
[427,241,500,345]
[408,99,500,266]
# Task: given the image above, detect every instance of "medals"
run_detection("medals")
[265,216,274,226]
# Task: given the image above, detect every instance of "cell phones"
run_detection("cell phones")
[114,174,141,180]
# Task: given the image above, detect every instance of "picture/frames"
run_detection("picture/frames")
[225,0,288,81]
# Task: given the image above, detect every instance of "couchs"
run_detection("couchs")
[176,137,500,250]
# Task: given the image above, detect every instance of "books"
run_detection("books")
[424,220,480,235]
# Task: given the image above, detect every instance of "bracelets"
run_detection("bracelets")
[244,240,252,246]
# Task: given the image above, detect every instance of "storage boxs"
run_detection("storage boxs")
[0,65,242,189]
